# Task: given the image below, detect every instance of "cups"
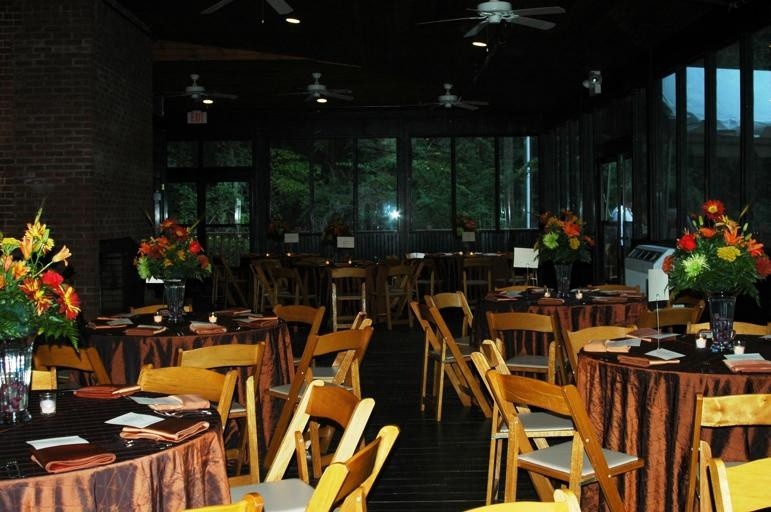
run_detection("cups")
[208,311,218,324]
[39,392,57,418]
[575,290,583,299]
[733,340,746,355]
[695,333,707,348]
[544,287,551,297]
[153,311,163,324]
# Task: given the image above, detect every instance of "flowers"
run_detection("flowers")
[138,220,218,281]
[323,214,352,245]
[659,199,771,296]
[264,217,289,243]
[529,207,587,263]
[451,215,481,252]
[1,206,93,354]
